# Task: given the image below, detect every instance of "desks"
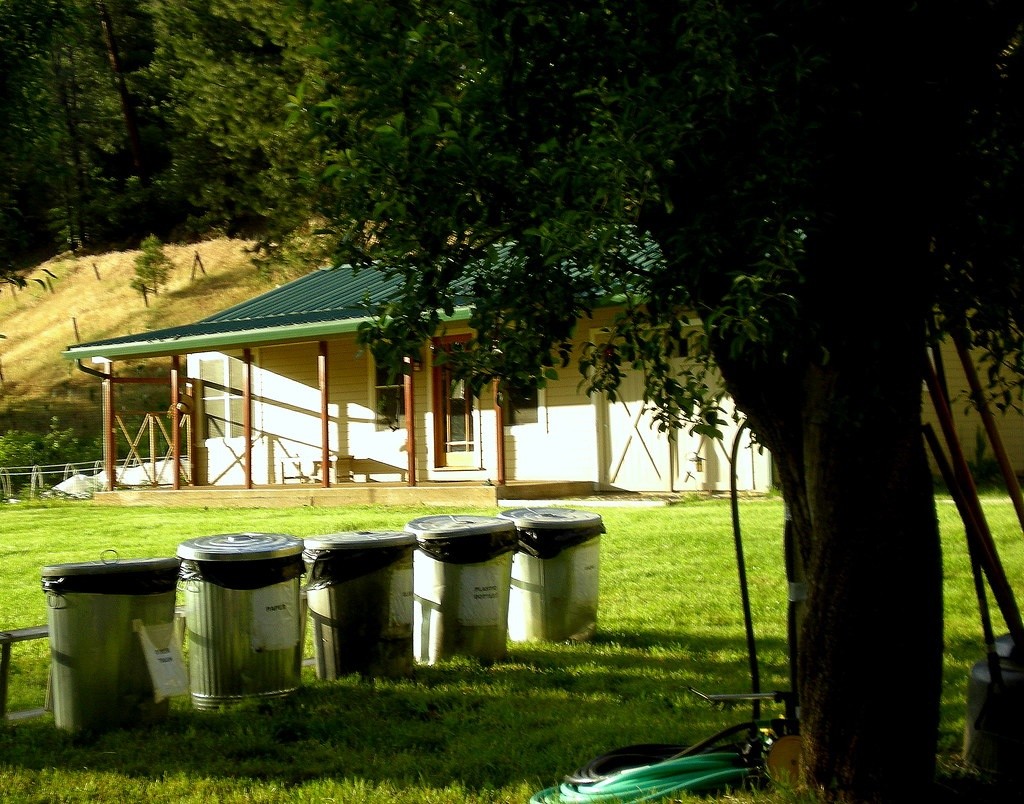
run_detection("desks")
[281,455,353,485]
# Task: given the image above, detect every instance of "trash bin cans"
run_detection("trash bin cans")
[176,532,306,713]
[302,530,420,683]
[405,514,518,666]
[500,507,605,643]
[41,550,181,740]
